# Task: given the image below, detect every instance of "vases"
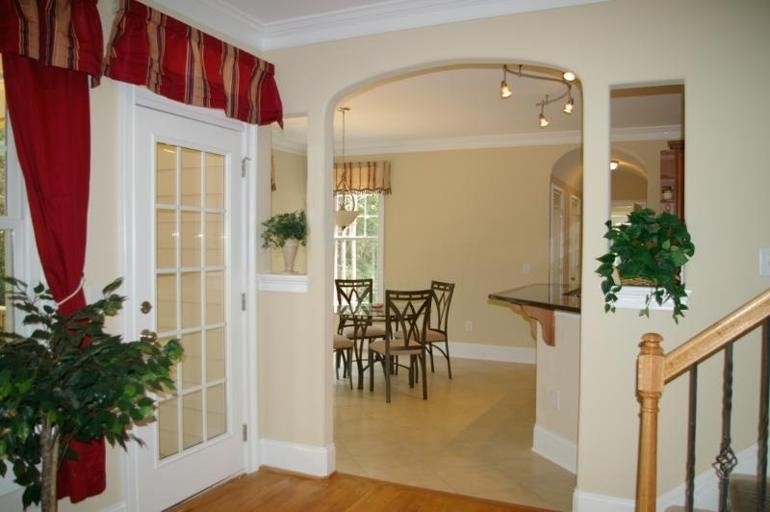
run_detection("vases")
[282,238,298,273]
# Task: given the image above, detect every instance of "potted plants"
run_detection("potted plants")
[591,201,695,325]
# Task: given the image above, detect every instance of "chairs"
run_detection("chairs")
[332,279,455,405]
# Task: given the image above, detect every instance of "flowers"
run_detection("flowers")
[258,211,307,249]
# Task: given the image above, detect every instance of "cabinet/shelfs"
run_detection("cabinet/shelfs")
[550,173,583,298]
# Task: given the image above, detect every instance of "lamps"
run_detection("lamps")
[498,62,577,130]
[332,108,360,231]
